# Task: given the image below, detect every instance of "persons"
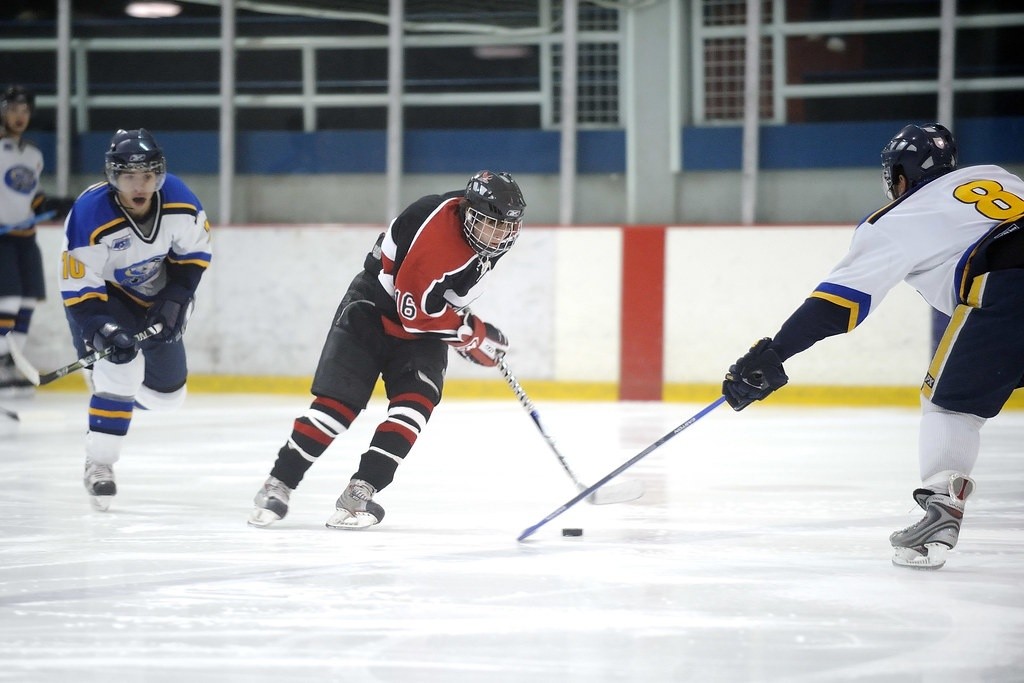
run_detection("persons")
[249,171,527,529]
[723,124,1023,572]
[59,127,212,514]
[0,86,77,419]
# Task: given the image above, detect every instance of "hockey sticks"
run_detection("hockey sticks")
[515,395,728,542]
[497,356,601,506]
[5,321,165,387]
[0,208,59,233]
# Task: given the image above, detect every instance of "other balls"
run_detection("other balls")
[561,528,584,537]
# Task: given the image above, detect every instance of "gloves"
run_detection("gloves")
[726,338,788,400]
[86,322,139,365]
[143,287,193,343]
[46,198,74,220]
[442,310,508,366]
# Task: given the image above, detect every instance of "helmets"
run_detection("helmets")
[880,122,959,190]
[105,127,166,174]
[3,85,36,106]
[460,170,527,226]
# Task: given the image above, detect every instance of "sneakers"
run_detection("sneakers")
[325,479,384,529]
[247,475,291,527]
[889,476,976,570]
[83,457,117,510]
